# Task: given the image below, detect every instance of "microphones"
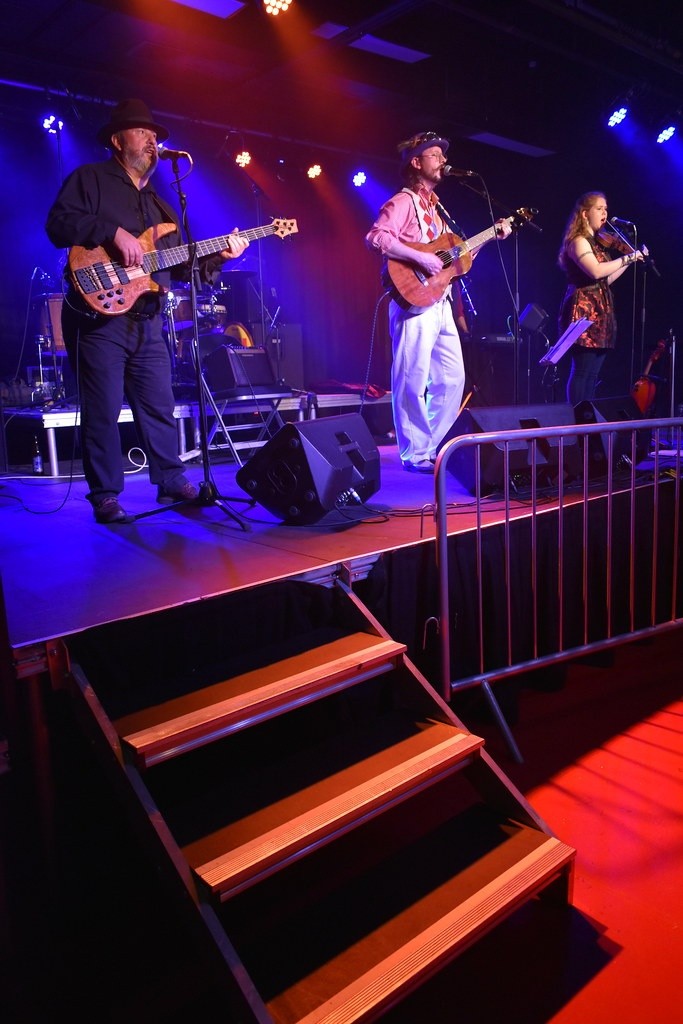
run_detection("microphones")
[158,147,189,161]
[443,165,478,178]
[611,217,634,226]
[38,268,50,278]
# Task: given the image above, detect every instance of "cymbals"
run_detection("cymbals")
[221,270,258,280]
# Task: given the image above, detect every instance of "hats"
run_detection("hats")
[400,132,449,169]
[96,98,169,151]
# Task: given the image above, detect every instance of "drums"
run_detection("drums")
[164,287,227,326]
[31,291,68,350]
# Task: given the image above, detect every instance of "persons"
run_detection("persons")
[556,192,649,405]
[46,98,250,523]
[363,132,512,474]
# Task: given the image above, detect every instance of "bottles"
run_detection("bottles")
[32,435,45,475]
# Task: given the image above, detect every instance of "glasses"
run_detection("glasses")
[420,132,441,144]
[419,152,447,161]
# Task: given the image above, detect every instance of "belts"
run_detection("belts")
[127,311,153,321]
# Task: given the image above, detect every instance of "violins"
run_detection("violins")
[632,340,667,413]
[595,229,638,254]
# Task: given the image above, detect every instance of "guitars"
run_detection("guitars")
[381,207,538,314]
[68,215,298,318]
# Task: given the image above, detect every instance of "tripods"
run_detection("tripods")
[14,276,72,410]
[126,159,256,532]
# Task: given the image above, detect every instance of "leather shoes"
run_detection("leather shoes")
[403,458,436,474]
[157,482,215,507]
[94,497,126,522]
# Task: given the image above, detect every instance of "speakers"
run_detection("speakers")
[234,413,380,525]
[203,271,305,392]
[436,396,649,498]
